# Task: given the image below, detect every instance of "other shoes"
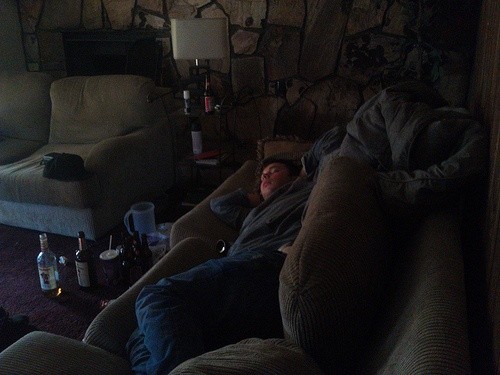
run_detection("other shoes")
[0,316,29,343]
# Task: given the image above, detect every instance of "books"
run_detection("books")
[195,151,228,166]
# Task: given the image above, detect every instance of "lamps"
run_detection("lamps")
[170,18,231,106]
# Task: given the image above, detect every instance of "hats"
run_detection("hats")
[43,153,95,182]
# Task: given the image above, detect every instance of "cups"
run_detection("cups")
[124,202,156,234]
[99,250,122,281]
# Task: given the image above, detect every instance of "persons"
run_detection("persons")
[125,126,347,375]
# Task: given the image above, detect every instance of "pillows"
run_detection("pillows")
[255,136,314,190]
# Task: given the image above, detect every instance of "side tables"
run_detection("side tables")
[159,101,240,210]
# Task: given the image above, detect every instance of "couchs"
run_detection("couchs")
[0,74,476,375]
[0,73,178,238]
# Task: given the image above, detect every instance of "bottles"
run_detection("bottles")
[75,231,96,291]
[36,232,61,297]
[203,74,215,115]
[118,231,152,286]
[191,122,203,155]
[183,90,192,115]
